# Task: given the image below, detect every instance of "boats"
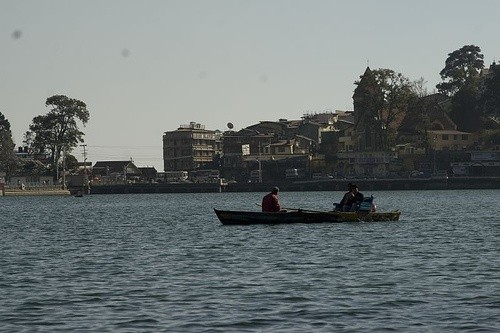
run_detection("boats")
[212,207,401,226]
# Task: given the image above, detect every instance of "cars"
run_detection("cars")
[410,171,424,177]
[312,173,334,180]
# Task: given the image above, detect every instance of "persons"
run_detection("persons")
[262,187,280,212]
[335,183,364,212]
